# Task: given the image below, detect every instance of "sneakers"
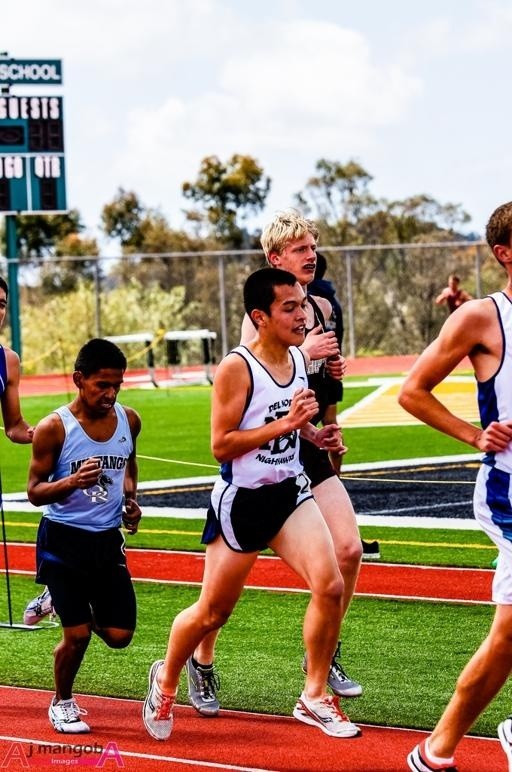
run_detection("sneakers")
[497,718,512,771]
[47,695,91,733]
[406,738,460,772]
[301,641,363,697]
[142,659,178,742]
[292,691,362,738]
[186,655,220,718]
[23,585,56,625]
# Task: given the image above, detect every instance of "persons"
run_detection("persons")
[0,277,34,442]
[142,268,361,743]
[187,212,364,716]
[308,254,344,472]
[398,201,512,772]
[434,274,472,312]
[24,339,141,734]
